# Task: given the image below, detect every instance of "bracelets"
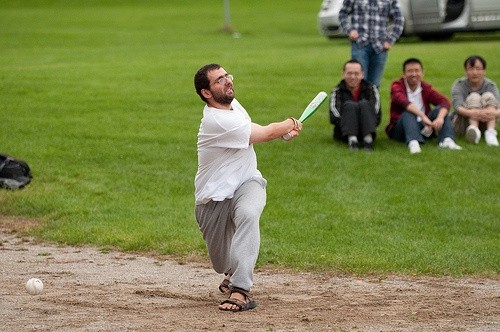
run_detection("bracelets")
[290,117,297,128]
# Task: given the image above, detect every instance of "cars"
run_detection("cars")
[318,0,500,42]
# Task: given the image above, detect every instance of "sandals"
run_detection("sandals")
[219,287,257,312]
[219,273,232,294]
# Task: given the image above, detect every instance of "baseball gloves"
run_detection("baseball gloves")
[0,154,32,191]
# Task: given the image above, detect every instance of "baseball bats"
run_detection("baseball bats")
[283,90,328,141]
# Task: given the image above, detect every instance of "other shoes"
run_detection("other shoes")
[440,139,461,150]
[361,141,372,151]
[410,143,421,154]
[349,142,358,151]
[485,130,498,146]
[466,126,480,144]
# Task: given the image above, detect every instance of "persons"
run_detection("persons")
[452,55,500,147]
[329,59,382,152]
[339,0,404,89]
[194,64,304,310]
[386,59,462,154]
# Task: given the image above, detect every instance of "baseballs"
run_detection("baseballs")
[26,278,44,295]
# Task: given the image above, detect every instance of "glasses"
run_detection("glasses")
[209,75,233,87]
